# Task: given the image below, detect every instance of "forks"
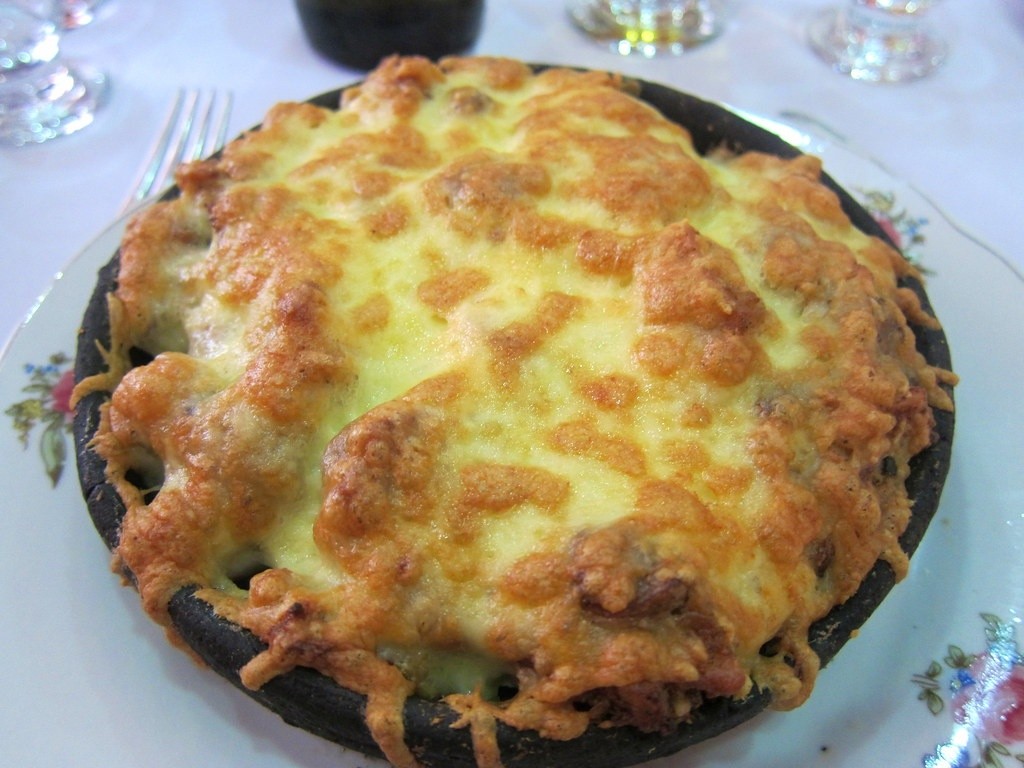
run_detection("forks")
[124,87,236,212]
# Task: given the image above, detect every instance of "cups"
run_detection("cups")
[805,0,949,82]
[0,1,114,147]
[566,0,719,53]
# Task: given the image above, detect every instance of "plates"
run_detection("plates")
[0,62,1023,768]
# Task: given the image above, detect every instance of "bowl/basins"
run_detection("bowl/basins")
[292,1,490,75]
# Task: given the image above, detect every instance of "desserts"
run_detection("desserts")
[63,49,957,768]
